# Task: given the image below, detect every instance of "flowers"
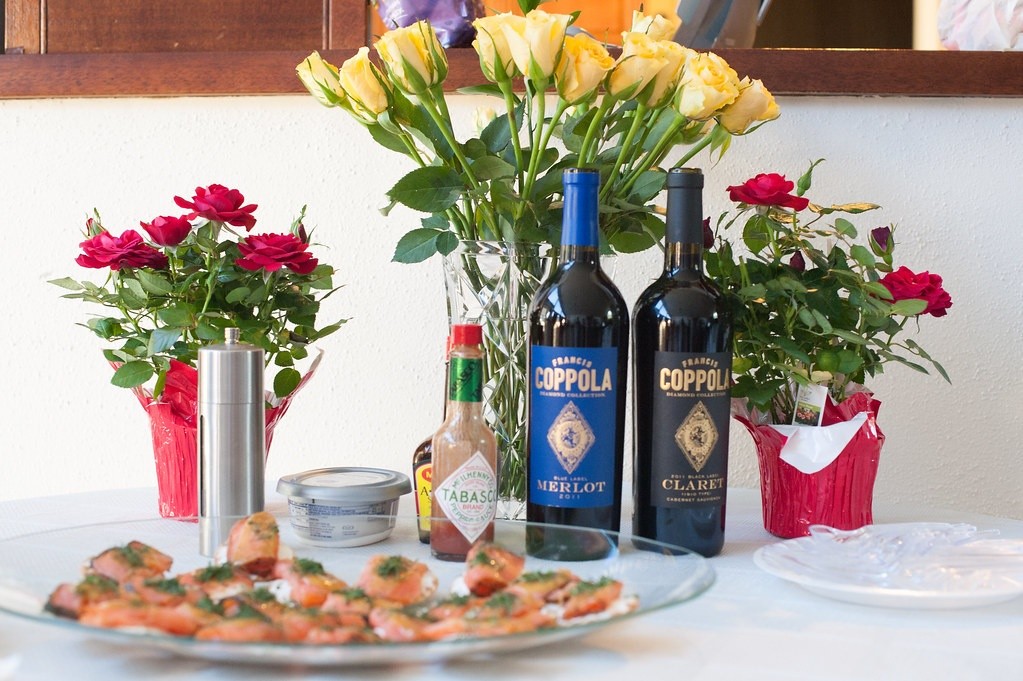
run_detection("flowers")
[702,157,953,426]
[44,182,354,399]
[293,0,782,266]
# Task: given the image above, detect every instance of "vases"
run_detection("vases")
[441,241,618,522]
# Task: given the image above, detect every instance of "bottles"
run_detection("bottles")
[631,166,735,559]
[524,167,630,561]
[412,323,497,562]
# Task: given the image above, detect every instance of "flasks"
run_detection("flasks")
[196,326,266,558]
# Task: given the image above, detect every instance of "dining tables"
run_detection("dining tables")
[0,487,1023,681]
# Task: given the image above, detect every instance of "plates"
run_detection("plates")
[752,536,1023,610]
[0,516,716,666]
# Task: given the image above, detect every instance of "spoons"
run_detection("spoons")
[808,523,1013,589]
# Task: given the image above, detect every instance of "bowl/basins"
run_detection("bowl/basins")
[275,467,413,547]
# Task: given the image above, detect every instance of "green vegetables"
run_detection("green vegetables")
[72,518,613,642]
